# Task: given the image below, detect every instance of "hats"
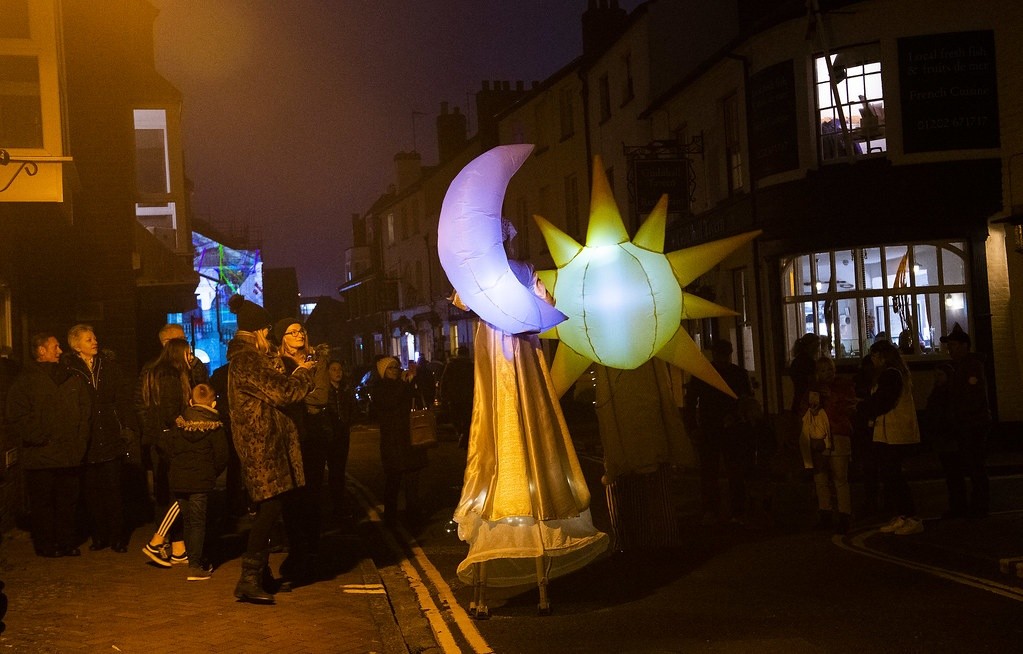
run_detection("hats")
[229,294,273,332]
[274,317,303,345]
[377,357,397,379]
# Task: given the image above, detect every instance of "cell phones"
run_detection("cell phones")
[305,353,314,362]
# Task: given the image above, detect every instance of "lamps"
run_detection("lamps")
[914,244,923,273]
[946,294,954,306]
[816,259,821,291]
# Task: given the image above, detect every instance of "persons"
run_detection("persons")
[372,347,474,529]
[449,210,611,588]
[791,321,994,532]
[7,294,351,603]
[683,341,773,529]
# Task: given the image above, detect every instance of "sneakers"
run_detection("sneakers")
[880,516,905,534]
[170,551,189,565]
[894,518,924,535]
[142,542,172,567]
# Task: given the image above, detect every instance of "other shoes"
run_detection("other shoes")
[88,540,109,551]
[110,541,128,553]
[736,501,757,514]
[944,502,965,517]
[187,567,211,581]
[701,510,719,527]
[37,538,62,557]
[967,502,992,520]
[203,560,214,571]
[63,538,81,555]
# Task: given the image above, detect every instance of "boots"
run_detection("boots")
[234,553,292,602]
[814,509,834,531]
[837,513,851,535]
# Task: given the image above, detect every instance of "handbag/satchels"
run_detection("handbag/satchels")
[811,448,833,476]
[409,394,438,447]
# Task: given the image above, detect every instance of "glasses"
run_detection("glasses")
[387,365,399,369]
[285,328,307,337]
[268,324,273,330]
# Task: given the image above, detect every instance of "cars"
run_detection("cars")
[343,358,473,439]
